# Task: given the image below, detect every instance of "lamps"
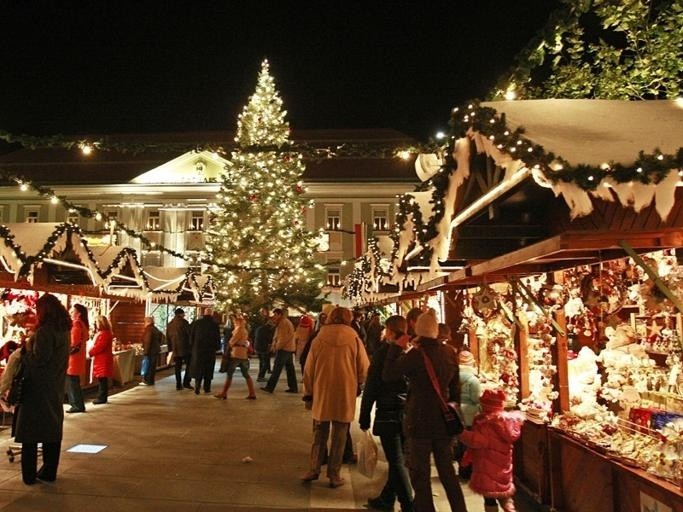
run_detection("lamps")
[414,153,446,183]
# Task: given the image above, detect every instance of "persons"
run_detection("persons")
[141,304,300,399]
[298,303,526,512]
[65,304,89,413]
[11,294,73,485]
[88,315,114,404]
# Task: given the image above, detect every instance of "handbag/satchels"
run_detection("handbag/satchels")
[141,356,149,376]
[8,363,25,404]
[442,401,465,436]
[231,346,248,360]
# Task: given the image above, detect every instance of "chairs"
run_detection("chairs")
[112,349,135,387]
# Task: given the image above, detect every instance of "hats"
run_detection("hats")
[144,317,153,328]
[479,390,507,409]
[459,351,475,364]
[174,308,184,314]
[415,308,439,339]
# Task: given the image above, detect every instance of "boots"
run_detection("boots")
[214,379,232,399]
[246,377,256,399]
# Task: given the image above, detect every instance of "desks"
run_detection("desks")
[514,408,682,512]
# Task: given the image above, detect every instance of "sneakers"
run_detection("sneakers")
[257,376,298,393]
[66,408,85,412]
[176,384,210,394]
[138,381,153,386]
[342,454,357,464]
[93,399,107,404]
[367,496,393,511]
[330,477,344,487]
[300,470,319,480]
